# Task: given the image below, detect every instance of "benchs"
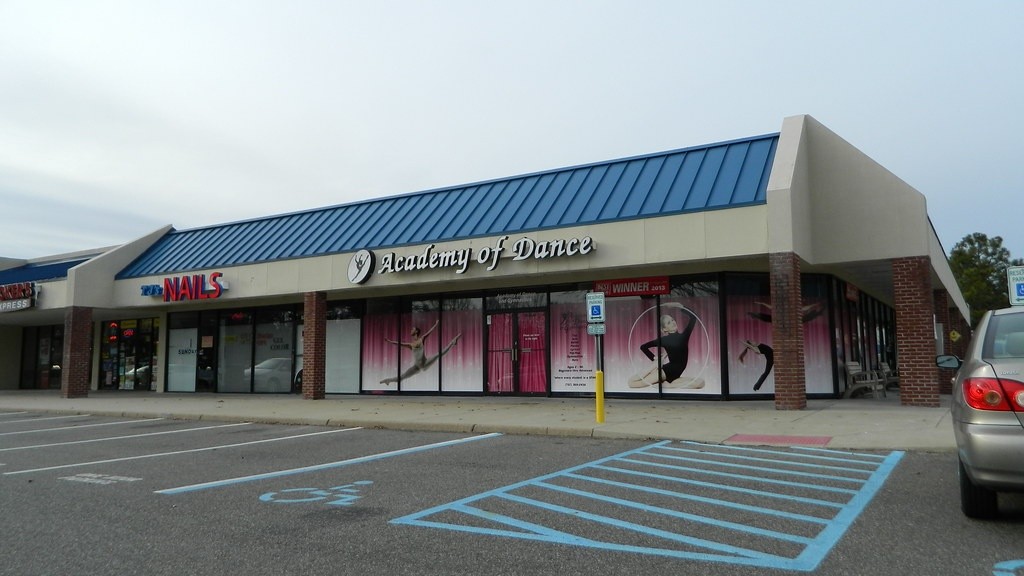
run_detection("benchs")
[843,361,887,399]
[879,363,898,377]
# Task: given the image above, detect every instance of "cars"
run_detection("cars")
[124,363,219,392]
[240,355,300,392]
[934,306,1024,521]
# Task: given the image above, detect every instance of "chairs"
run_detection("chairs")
[1006,332,1024,356]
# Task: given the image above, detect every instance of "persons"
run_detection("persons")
[628,303,705,389]
[380,319,462,385]
[739,339,773,391]
